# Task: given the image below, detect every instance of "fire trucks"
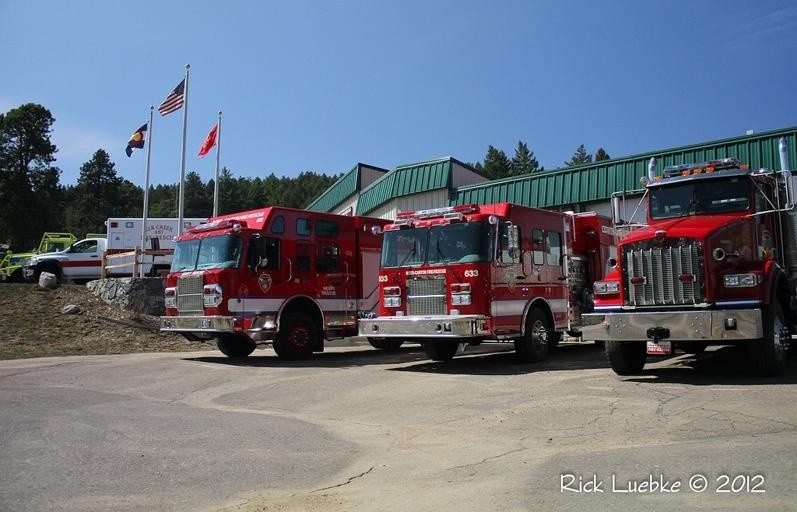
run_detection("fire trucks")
[356,197,647,363]
[156,205,395,362]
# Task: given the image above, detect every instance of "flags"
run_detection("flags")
[195,122,219,157]
[157,76,185,117]
[125,121,148,158]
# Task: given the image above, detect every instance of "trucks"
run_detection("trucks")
[22,216,211,286]
[577,132,797,377]
